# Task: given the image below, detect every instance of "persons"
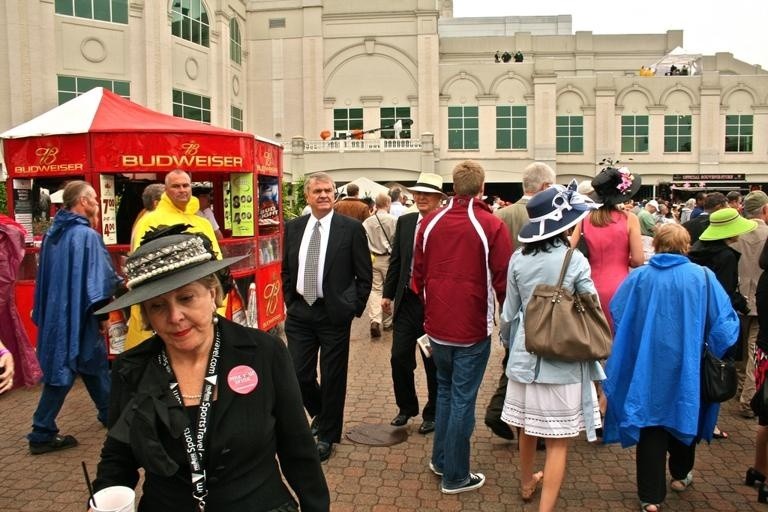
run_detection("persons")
[0,341,15,394]
[639,65,646,76]
[501,51,511,63]
[388,185,410,227]
[495,50,501,62]
[281,172,370,457]
[743,236,767,503]
[126,170,229,349]
[670,65,689,75]
[682,191,727,244]
[657,197,682,223]
[514,51,523,62]
[123,183,168,281]
[0,222,44,388]
[359,193,401,338]
[414,166,515,495]
[492,161,555,453]
[646,67,653,76]
[381,172,448,435]
[496,184,613,512]
[24,180,131,455]
[688,208,758,440]
[567,166,646,437]
[635,200,660,264]
[333,184,370,224]
[729,190,768,420]
[690,192,707,220]
[627,199,634,212]
[85,232,332,512]
[603,223,741,511]
[191,186,224,239]
[682,197,697,224]
[726,190,742,209]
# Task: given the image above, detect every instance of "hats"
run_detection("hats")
[92,226,251,316]
[407,173,449,199]
[515,182,597,244]
[700,209,756,242]
[648,199,662,213]
[743,190,768,211]
[592,167,641,206]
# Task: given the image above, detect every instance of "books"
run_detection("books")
[416,334,433,359]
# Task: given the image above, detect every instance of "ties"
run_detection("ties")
[305,223,319,306]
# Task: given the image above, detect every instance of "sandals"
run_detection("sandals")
[522,470,544,502]
[673,472,694,490]
[713,427,729,438]
[641,497,661,512]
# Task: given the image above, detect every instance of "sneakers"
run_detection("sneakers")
[441,472,487,495]
[379,335,383,338]
[370,323,383,341]
[31,434,77,453]
[428,457,445,478]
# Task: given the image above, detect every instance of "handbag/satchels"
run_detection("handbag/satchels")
[704,269,737,404]
[524,247,612,360]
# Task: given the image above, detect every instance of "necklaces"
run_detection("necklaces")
[180,394,202,399]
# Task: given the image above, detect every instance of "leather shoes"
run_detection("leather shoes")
[418,417,438,435]
[316,438,334,460]
[485,413,515,442]
[310,415,321,435]
[390,412,417,428]
[746,467,768,503]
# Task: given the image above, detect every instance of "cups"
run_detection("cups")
[86,484,137,511]
